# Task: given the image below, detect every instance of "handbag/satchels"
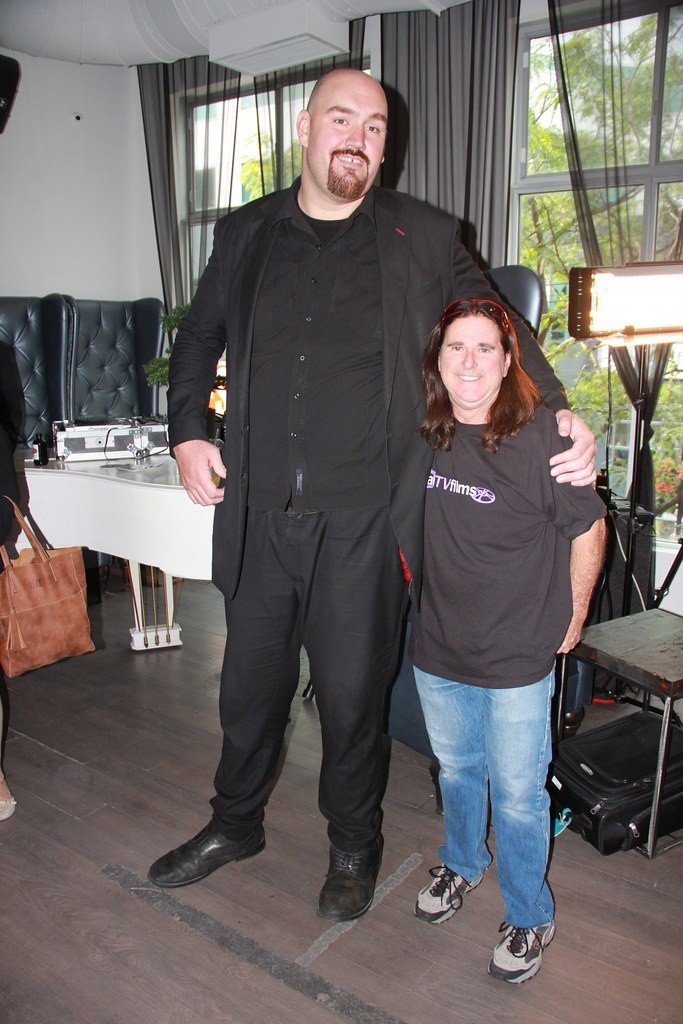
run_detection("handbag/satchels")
[0,494,97,679]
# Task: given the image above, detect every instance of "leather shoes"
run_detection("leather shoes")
[317,832,384,923]
[147,820,266,889]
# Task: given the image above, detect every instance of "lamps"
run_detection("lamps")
[563,260,681,346]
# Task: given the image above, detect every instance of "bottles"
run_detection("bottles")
[33,433,48,466]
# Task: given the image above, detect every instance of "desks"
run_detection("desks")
[559,605,682,858]
[18,440,216,651]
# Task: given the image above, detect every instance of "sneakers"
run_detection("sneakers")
[486,919,556,985]
[413,861,484,924]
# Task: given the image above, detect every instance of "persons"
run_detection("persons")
[0,341,24,823]
[411,295,609,986]
[147,68,598,926]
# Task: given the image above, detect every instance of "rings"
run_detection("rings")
[591,461,596,469]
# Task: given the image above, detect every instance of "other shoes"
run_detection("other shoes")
[0,796,18,821]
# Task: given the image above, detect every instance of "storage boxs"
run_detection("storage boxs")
[552,708,683,854]
